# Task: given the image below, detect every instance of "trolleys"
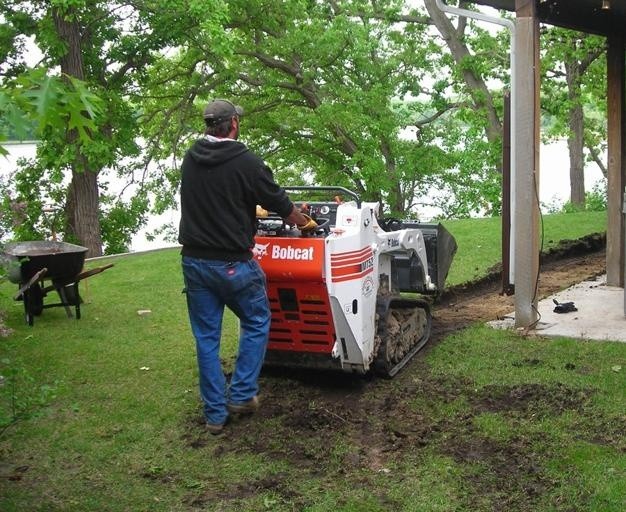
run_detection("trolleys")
[5,240,114,326]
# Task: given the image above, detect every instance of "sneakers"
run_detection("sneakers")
[205,396,258,435]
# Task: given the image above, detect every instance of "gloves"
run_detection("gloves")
[256,205,269,217]
[297,213,318,231]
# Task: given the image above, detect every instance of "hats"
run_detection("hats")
[204,99,242,122]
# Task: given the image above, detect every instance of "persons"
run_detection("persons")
[178,100,321,434]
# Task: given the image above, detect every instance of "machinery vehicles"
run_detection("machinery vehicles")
[239,186,457,380]
